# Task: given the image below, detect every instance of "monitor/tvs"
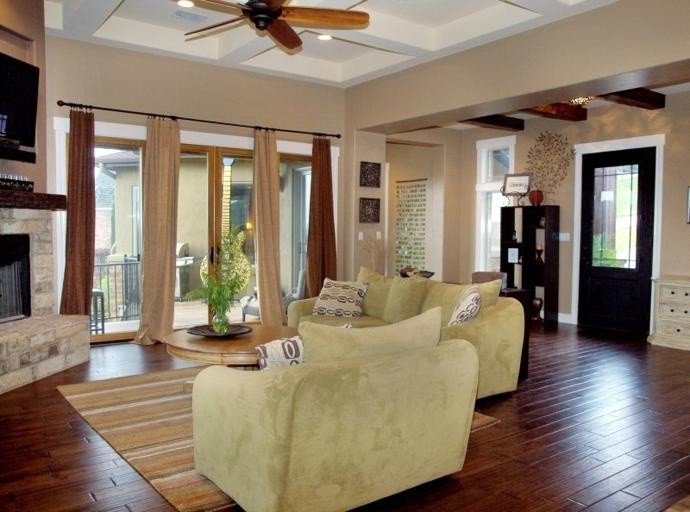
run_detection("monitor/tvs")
[0,52,40,164]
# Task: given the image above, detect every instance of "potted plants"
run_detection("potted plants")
[183,226,246,334]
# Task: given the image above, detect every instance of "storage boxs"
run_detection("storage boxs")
[471,271,508,290]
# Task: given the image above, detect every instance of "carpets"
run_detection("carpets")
[56,363,502,512]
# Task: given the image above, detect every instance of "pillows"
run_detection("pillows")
[312,277,369,318]
[297,306,444,364]
[444,278,503,326]
[355,265,394,318]
[253,323,356,370]
[421,282,469,326]
[382,274,428,324]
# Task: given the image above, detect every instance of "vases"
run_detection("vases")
[535,249,544,269]
[540,216,546,227]
[531,297,543,321]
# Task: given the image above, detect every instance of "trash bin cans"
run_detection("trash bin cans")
[175,243,195,301]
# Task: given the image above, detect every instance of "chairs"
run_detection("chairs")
[192,338,479,512]
[240,288,260,321]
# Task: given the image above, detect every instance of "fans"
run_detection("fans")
[170,0,370,56]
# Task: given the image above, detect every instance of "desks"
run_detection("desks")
[500,287,529,381]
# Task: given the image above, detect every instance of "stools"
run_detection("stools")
[89,290,105,334]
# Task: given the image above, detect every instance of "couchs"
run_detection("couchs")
[287,277,526,400]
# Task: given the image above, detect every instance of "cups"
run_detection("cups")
[529,190,544,205]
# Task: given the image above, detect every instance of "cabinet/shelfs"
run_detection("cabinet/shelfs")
[646,273,690,351]
[499,204,560,334]
